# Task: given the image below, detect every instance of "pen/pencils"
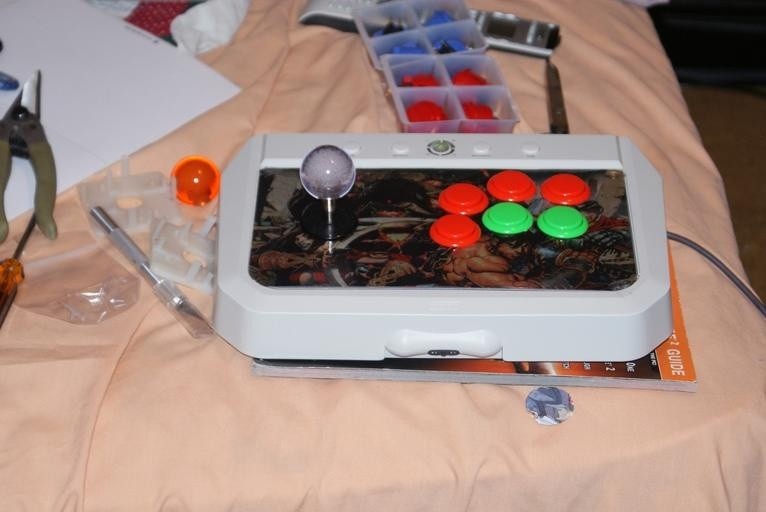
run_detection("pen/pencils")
[88,205,205,324]
[544,60,570,135]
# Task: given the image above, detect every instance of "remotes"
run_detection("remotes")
[296,0,562,60]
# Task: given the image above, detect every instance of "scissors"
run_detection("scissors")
[0,68,57,246]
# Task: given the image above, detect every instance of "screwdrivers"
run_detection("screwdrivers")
[0,212,38,329]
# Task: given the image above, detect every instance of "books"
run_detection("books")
[250,238,700,395]
